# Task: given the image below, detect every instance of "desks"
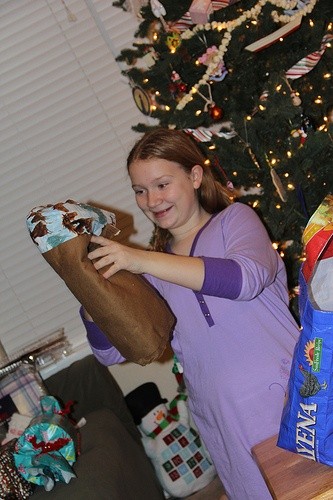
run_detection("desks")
[252,432,332,500]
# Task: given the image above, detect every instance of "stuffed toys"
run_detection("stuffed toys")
[123,381,216,497]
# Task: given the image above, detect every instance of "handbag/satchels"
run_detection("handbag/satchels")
[273,193,332,471]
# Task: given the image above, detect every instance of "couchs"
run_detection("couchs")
[0,355,166,500]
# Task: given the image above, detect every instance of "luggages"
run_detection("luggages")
[0,358,49,428]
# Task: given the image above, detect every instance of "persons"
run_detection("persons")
[79,128,300,500]
